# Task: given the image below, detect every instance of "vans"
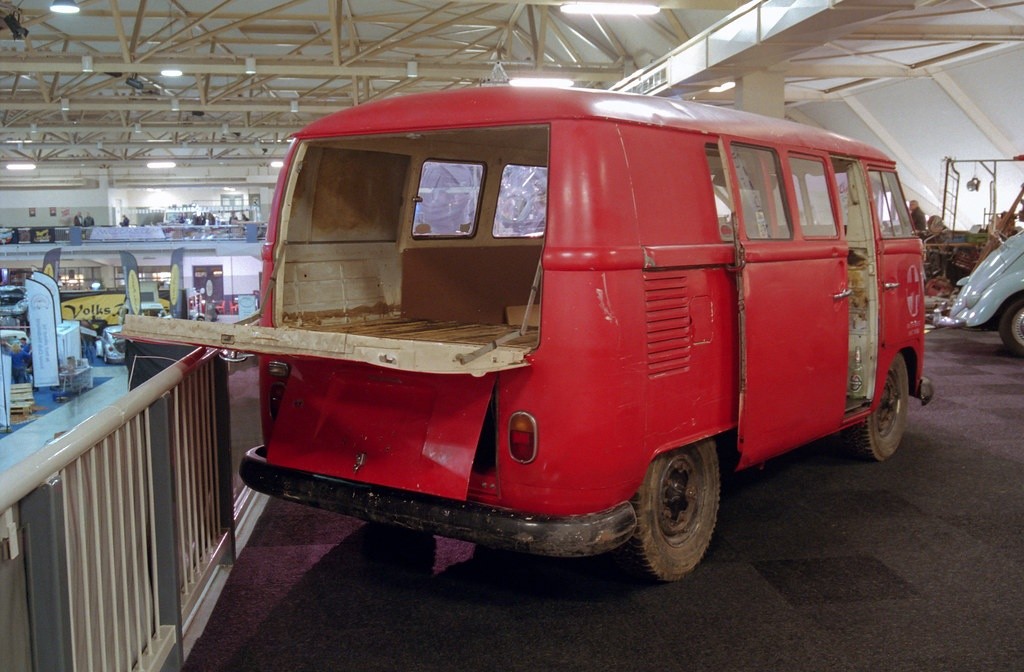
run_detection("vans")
[124,88,935,583]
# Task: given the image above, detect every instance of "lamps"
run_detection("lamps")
[0,0,739,176]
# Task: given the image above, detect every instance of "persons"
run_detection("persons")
[83,212,94,227]
[0,337,40,392]
[73,212,84,226]
[79,317,97,367]
[909,199,927,238]
[120,214,130,227]
[177,210,250,225]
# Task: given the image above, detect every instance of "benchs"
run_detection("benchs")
[10,383,34,419]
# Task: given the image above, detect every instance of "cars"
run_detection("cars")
[937,225,1023,360]
[100,325,126,364]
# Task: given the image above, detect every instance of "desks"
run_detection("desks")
[58,366,91,396]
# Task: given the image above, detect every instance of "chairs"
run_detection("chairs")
[202,299,238,315]
